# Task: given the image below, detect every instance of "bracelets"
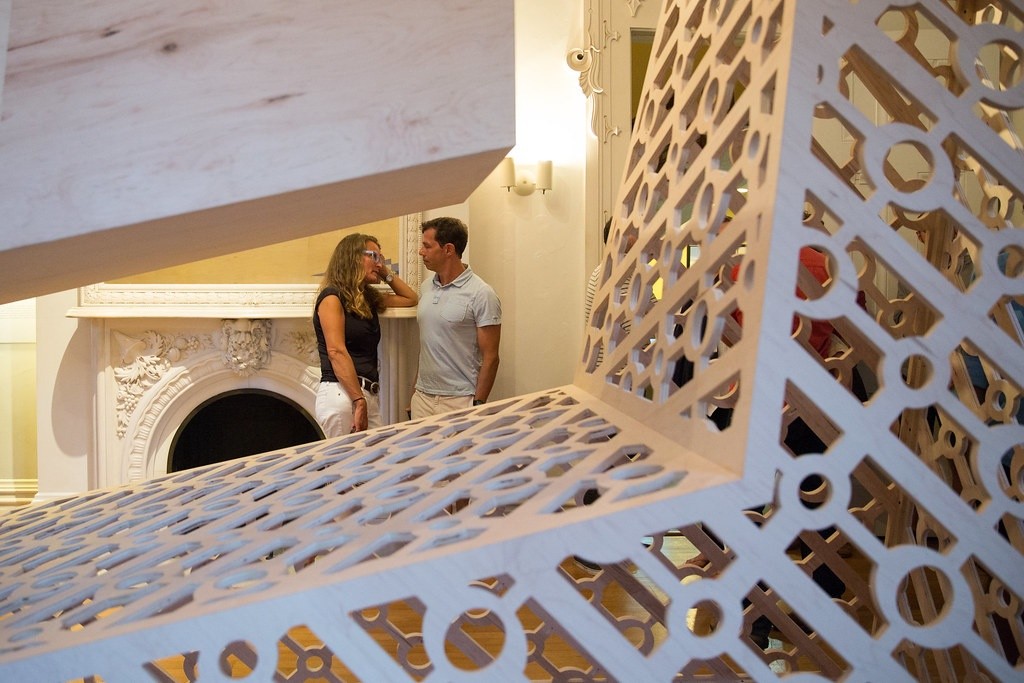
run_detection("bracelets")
[352,397,366,404]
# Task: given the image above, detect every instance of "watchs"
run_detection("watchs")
[473,399,486,406]
[384,271,396,284]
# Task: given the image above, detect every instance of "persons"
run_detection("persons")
[406,217,501,516]
[304,233,418,440]
[575,204,1023,668]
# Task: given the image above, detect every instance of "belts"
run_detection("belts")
[357,376,380,395]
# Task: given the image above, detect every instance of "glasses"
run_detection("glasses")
[364,250,385,261]
